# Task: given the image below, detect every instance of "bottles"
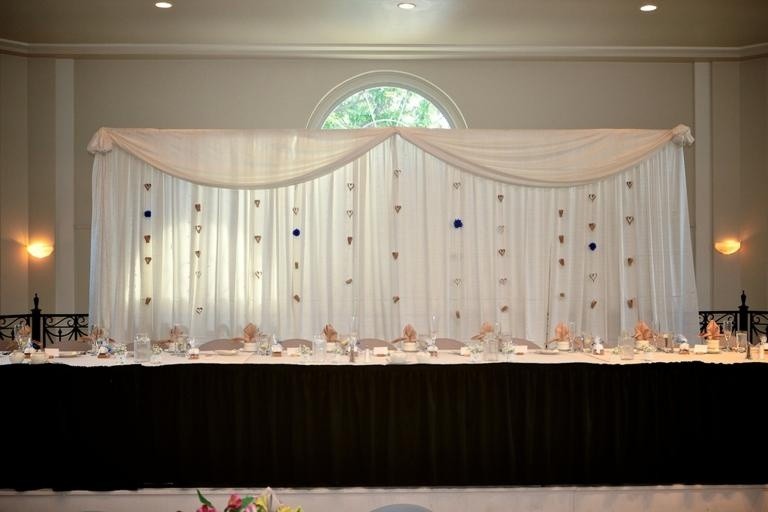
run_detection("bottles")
[745,343,765,360]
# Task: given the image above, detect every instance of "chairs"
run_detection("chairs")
[0,334,768,353]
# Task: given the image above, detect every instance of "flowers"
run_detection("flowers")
[175,490,306,511]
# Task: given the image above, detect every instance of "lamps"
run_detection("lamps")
[714,239,743,257]
[24,241,54,258]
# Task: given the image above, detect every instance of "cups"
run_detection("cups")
[737,331,748,351]
[10,353,26,364]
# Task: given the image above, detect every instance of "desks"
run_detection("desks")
[2,349,767,488]
